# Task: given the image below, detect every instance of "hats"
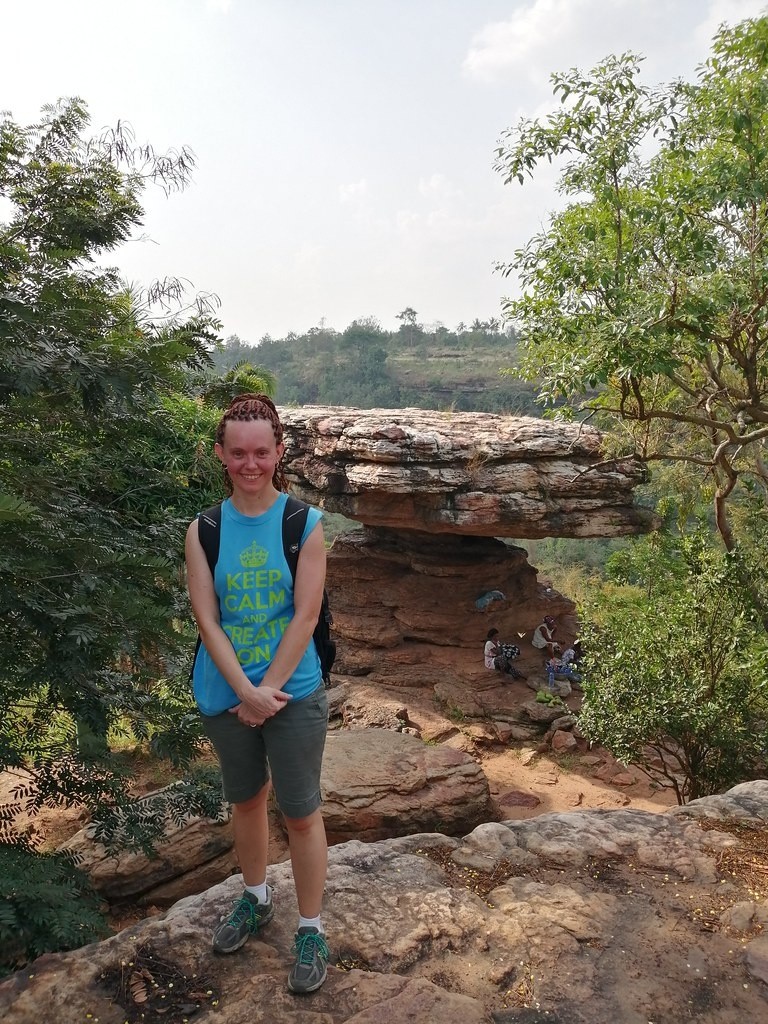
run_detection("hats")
[487,628,499,638]
[543,615,554,623]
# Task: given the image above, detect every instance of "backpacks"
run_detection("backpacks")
[198,495,336,688]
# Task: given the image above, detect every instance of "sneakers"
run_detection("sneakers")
[212,884,274,953]
[288,926,330,993]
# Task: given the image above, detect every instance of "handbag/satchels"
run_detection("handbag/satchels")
[502,643,520,659]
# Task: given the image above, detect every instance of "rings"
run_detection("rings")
[249,724,257,727]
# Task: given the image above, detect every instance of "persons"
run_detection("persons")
[484,628,520,679]
[182,394,335,994]
[562,642,584,668]
[532,615,565,660]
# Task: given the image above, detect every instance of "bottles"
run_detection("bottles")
[549,671,555,687]
